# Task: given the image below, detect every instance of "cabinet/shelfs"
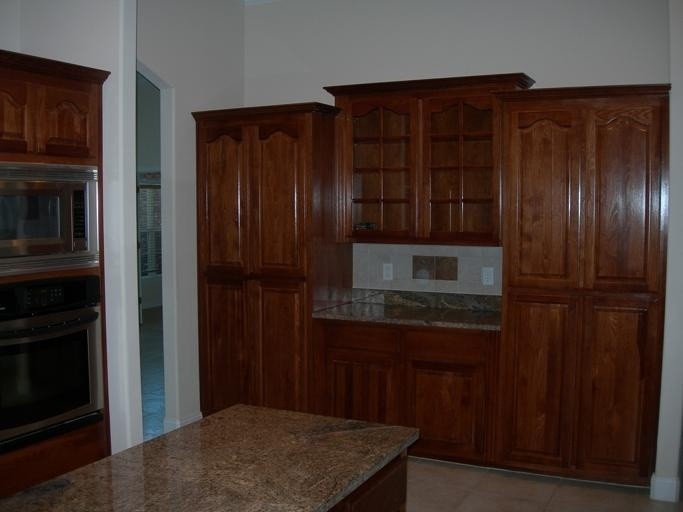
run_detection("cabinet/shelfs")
[191,102,343,417]
[323,73,535,248]
[0,66,103,166]
[313,315,500,468]
[0,421,111,501]
[493,83,672,489]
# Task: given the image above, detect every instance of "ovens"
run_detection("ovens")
[0,274,107,446]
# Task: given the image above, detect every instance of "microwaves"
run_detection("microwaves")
[0,159,101,277]
